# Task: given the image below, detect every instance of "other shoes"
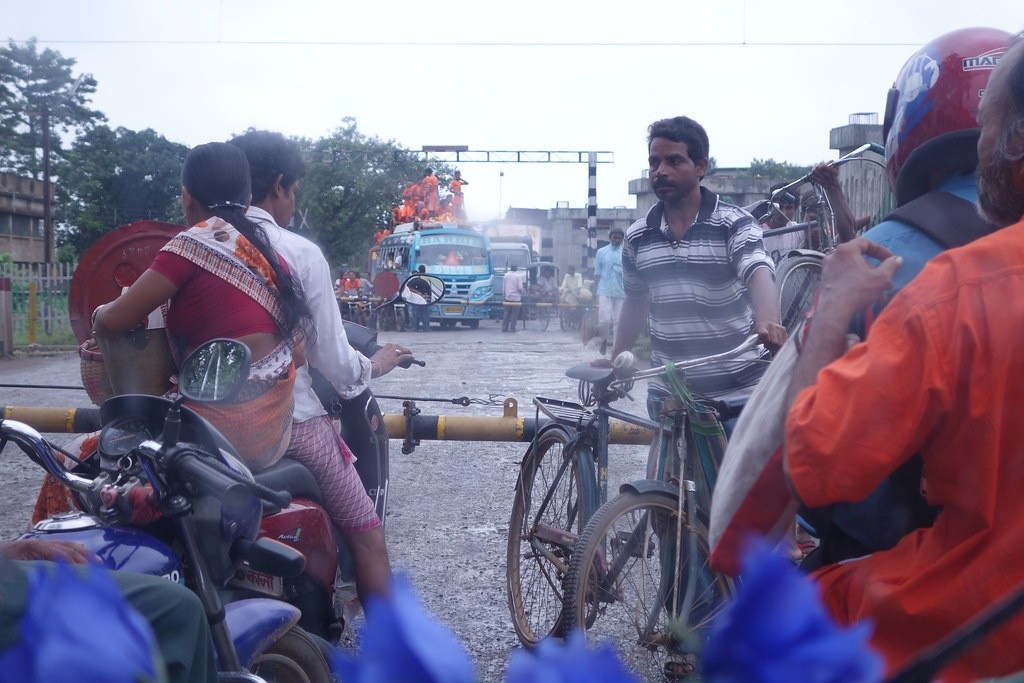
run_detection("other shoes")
[501,328,508,332]
[509,329,518,333]
[599,344,606,355]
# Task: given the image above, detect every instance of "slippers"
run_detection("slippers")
[662,652,698,679]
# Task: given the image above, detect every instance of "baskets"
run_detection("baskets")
[77,331,112,406]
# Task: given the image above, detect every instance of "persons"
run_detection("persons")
[589,117,790,681]
[534,267,558,298]
[369,247,485,270]
[372,166,469,244]
[560,265,582,301]
[755,159,857,275]
[334,269,376,305]
[499,265,524,332]
[226,130,415,631]
[593,228,625,356]
[29,142,305,547]
[782,29,1024,683]
[401,277,432,304]
[0,538,220,683]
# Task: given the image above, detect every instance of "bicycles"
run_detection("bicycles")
[560,288,583,332]
[739,142,887,337]
[506,333,772,683]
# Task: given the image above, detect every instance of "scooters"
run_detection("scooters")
[253,273,445,646]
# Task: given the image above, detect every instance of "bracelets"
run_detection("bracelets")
[91,303,106,325]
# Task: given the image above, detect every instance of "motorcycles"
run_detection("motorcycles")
[0,338,330,683]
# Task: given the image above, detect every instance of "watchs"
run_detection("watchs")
[370,359,382,378]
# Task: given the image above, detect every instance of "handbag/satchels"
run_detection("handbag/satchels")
[90,303,178,398]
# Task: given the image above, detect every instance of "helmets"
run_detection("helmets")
[881,25,1024,209]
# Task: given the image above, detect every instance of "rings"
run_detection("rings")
[395,348,402,356]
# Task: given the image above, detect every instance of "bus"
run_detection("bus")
[375,221,494,328]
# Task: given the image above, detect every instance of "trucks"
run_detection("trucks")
[488,243,531,318]
